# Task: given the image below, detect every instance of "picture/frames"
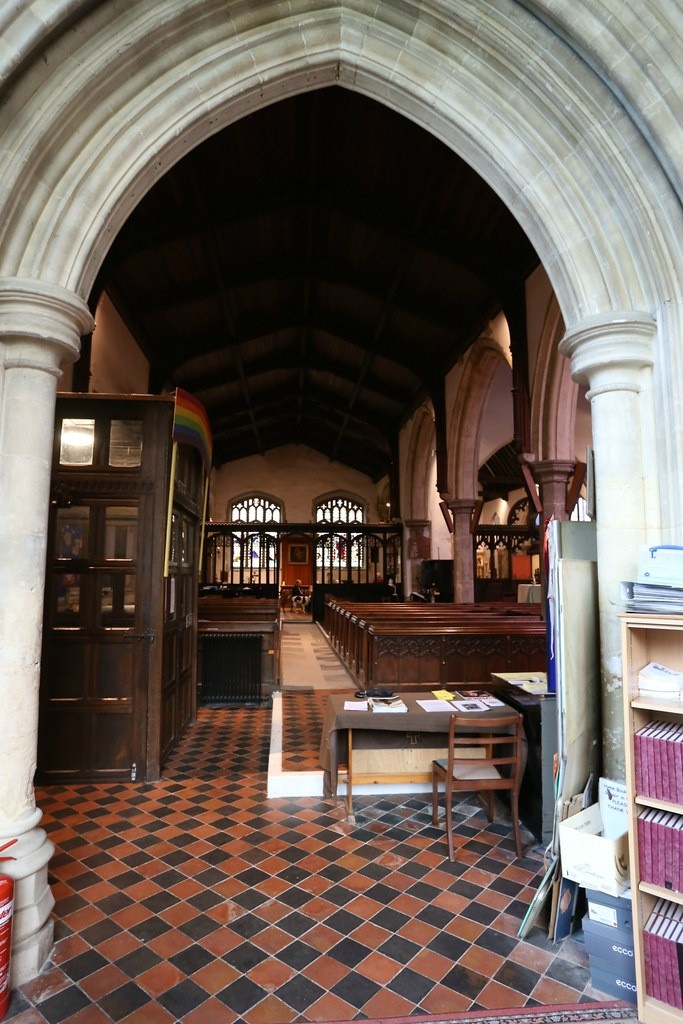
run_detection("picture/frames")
[288,543,310,566]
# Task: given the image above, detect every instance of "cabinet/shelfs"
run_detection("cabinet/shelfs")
[620,613,683,1024]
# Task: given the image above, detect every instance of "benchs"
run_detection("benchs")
[196,596,282,690]
[324,596,548,691]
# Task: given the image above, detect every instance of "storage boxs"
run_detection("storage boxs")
[586,889,633,935]
[557,801,630,897]
[582,911,634,967]
[588,953,637,1005]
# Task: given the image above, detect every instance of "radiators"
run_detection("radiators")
[196,633,269,707]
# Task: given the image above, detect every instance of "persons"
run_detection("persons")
[291,579,309,612]
[326,572,336,584]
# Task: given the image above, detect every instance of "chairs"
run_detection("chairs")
[432,713,525,862]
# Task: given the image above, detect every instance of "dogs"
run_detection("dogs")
[291,596,311,614]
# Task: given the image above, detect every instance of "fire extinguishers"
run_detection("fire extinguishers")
[0,839,18,1018]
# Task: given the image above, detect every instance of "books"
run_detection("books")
[367,695,408,713]
[631,718,683,1011]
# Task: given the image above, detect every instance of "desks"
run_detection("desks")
[321,690,522,814]
